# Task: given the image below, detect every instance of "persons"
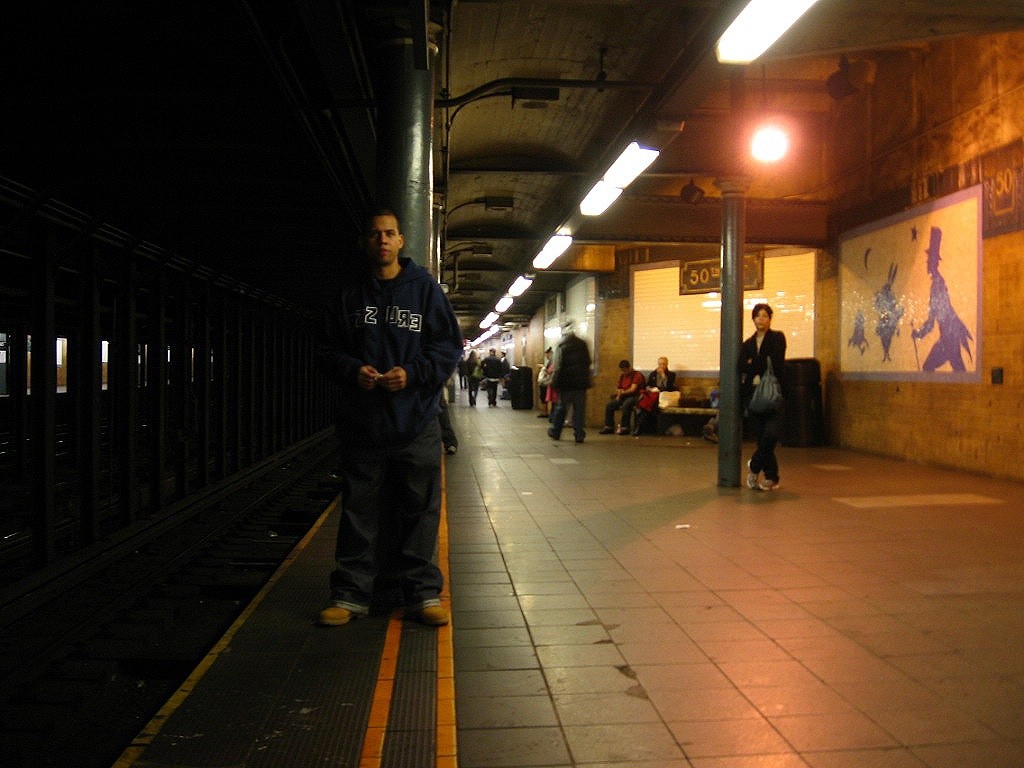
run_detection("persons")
[533,322,677,444]
[315,209,510,627]
[702,305,786,492]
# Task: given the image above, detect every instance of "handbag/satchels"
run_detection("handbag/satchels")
[657,390,681,409]
[748,356,782,412]
[537,367,553,384]
[472,359,481,380]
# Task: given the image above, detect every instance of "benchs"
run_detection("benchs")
[611,374,720,436]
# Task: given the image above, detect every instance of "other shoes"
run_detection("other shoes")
[448,446,457,454]
[599,428,614,434]
[538,414,548,418]
[575,434,584,443]
[617,430,629,435]
[632,428,643,435]
[489,401,496,406]
[417,605,449,626]
[746,459,760,488]
[319,607,355,624]
[547,429,558,440]
[759,479,781,490]
